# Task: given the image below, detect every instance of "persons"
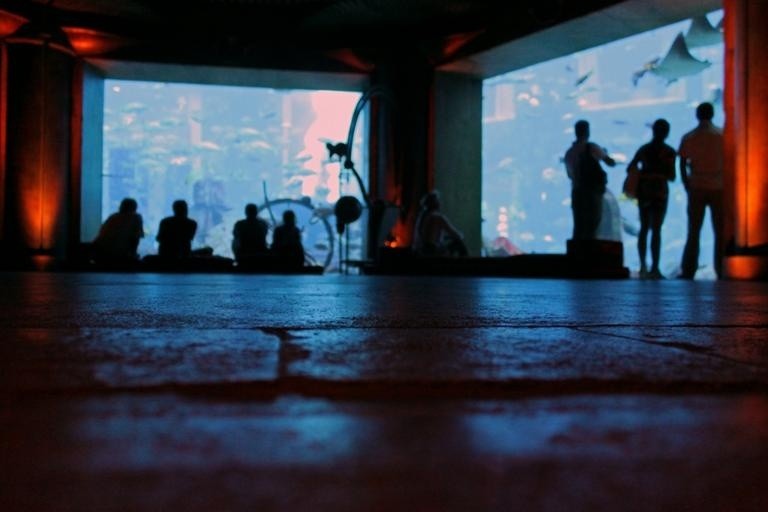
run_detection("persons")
[233,203,303,267]
[414,192,468,277]
[155,201,197,262]
[627,118,677,282]
[679,101,722,278]
[96,197,144,264]
[564,120,616,240]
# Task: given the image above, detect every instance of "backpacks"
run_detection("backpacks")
[573,142,605,180]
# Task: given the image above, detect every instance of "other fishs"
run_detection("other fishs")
[479,16,726,278]
[103,80,367,274]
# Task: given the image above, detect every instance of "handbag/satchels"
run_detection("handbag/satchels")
[622,163,642,199]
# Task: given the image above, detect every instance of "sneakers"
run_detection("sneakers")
[638,270,666,280]
[677,269,693,280]
